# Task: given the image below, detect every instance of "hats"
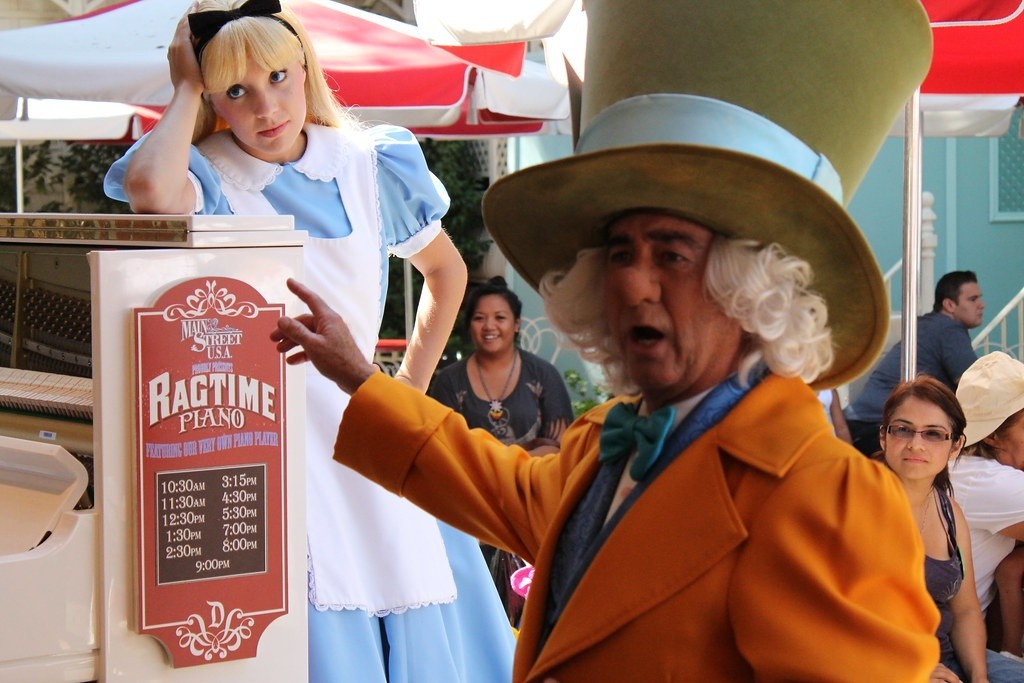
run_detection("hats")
[956,350,1024,447]
[481,0,934,391]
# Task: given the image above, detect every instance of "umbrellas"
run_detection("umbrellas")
[886,0,1024,379]
[0,0,569,349]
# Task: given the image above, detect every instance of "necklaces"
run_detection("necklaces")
[919,502,927,533]
[476,354,516,421]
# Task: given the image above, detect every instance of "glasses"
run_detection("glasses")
[882,423,957,442]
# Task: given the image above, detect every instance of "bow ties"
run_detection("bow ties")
[599,402,675,483]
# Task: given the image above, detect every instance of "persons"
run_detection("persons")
[424,276,571,632]
[268,91,939,683]
[947,352,1024,665]
[814,389,852,445]
[103,1,516,683]
[871,377,1024,683]
[843,270,984,459]
